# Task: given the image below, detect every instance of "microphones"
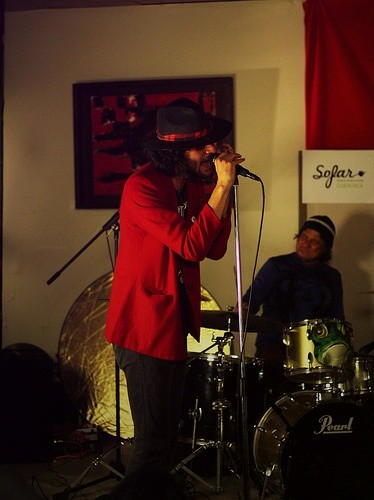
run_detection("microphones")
[207,154,260,180]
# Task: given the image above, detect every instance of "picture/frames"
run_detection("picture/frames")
[72,76,235,210]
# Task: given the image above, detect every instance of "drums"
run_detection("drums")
[253,389,374,500]
[337,356,374,400]
[184,352,265,418]
[282,318,355,384]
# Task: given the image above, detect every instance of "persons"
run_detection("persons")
[231,215,345,358]
[105,98,246,475]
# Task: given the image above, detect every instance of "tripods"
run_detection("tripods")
[173,342,254,493]
[49,212,129,492]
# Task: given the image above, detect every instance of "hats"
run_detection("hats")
[150,96,232,150]
[302,215,337,248]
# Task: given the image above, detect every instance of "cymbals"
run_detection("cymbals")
[200,310,267,333]
